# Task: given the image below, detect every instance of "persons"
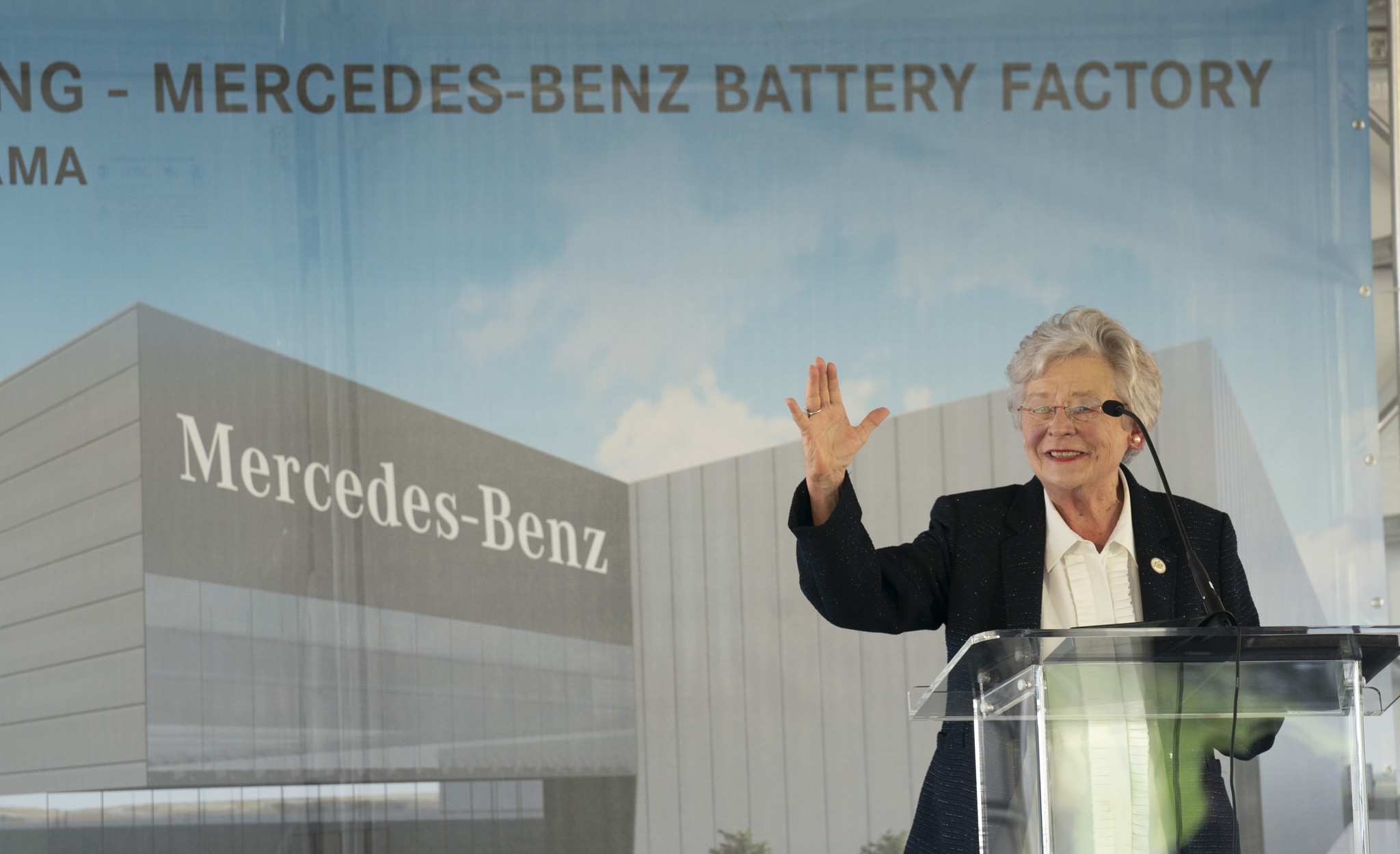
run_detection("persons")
[784,306,1285,854]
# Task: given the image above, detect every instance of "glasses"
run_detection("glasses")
[1017,394,1129,425]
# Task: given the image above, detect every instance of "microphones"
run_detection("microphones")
[1102,399,1230,629]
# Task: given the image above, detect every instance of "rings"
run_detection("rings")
[804,407,822,419]
